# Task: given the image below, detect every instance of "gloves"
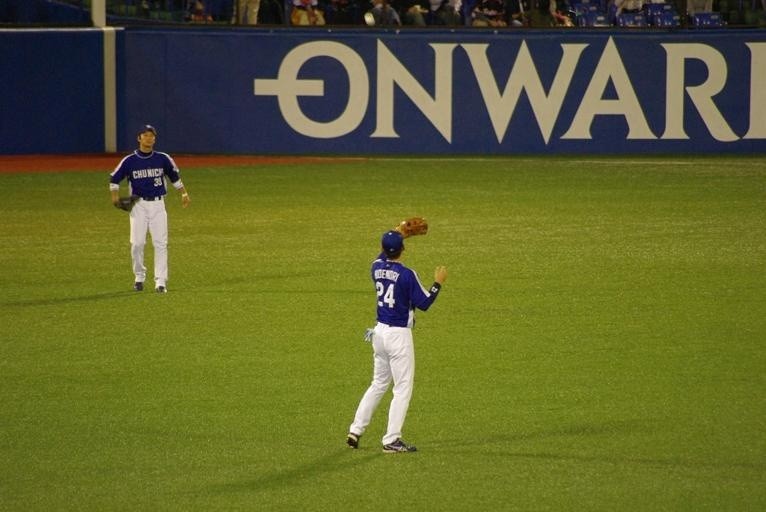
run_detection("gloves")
[364,329,374,345]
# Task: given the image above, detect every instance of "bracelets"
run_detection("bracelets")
[182,192,187,197]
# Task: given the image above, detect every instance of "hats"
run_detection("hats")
[138,124,154,135]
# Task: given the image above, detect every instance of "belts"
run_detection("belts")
[388,325,399,328]
[144,196,162,200]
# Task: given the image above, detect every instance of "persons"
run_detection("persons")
[346,229,447,454]
[109,124,191,292]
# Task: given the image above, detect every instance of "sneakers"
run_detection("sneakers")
[155,287,166,293]
[347,433,360,448]
[133,282,143,291]
[383,438,416,453]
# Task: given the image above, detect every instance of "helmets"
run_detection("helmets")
[381,231,403,251]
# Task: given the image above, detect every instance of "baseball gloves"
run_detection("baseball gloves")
[397,217,428,237]
[115,196,140,212]
